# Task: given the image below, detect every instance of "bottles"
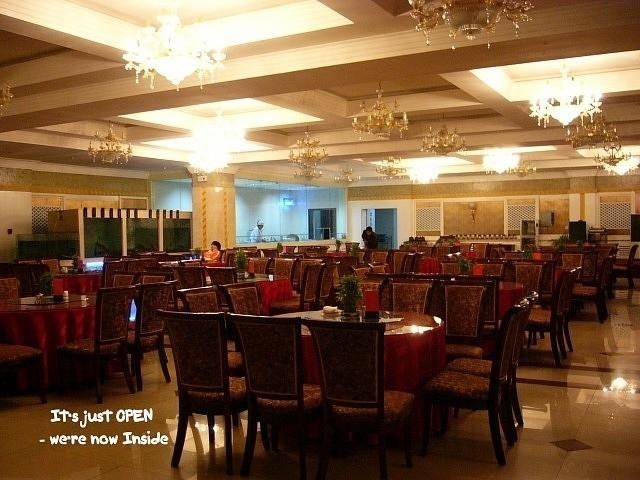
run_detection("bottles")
[63,291,69,303]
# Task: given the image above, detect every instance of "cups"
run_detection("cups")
[81,295,86,301]
[82,301,86,308]
[269,275,273,281]
[62,267,68,274]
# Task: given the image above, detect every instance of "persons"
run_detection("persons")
[362,230,369,249]
[248,220,265,242]
[365,226,378,249]
[200,240,222,264]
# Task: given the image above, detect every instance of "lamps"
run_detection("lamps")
[594,148,632,167]
[375,155,407,182]
[508,159,538,178]
[528,61,604,127]
[288,126,328,178]
[418,111,469,157]
[88,121,131,165]
[408,0,533,51]
[563,113,623,147]
[352,82,408,139]
[122,3,228,92]
[330,168,361,186]
[0,79,17,117]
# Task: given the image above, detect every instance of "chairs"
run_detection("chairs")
[0,241,640,480]
